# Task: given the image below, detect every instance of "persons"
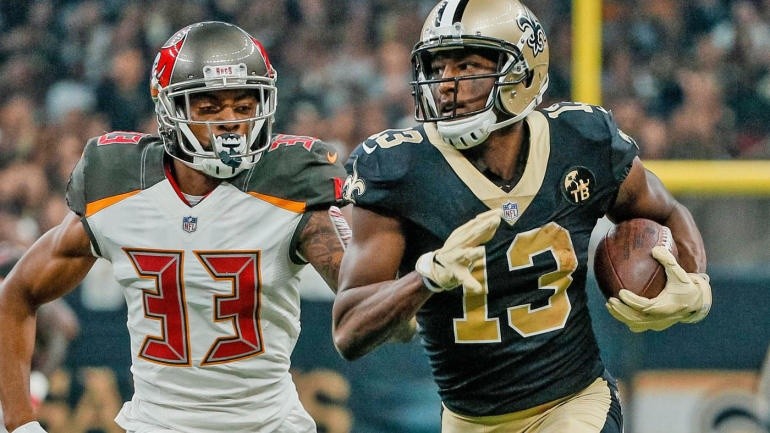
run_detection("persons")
[0,0,429,266]
[331,0,712,433]
[0,21,420,433]
[601,0,770,160]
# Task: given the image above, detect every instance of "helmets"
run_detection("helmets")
[410,0,548,150]
[148,22,278,179]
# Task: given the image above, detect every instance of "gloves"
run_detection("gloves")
[602,249,713,333]
[415,209,507,296]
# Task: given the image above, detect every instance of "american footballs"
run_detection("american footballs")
[592,217,680,306]
